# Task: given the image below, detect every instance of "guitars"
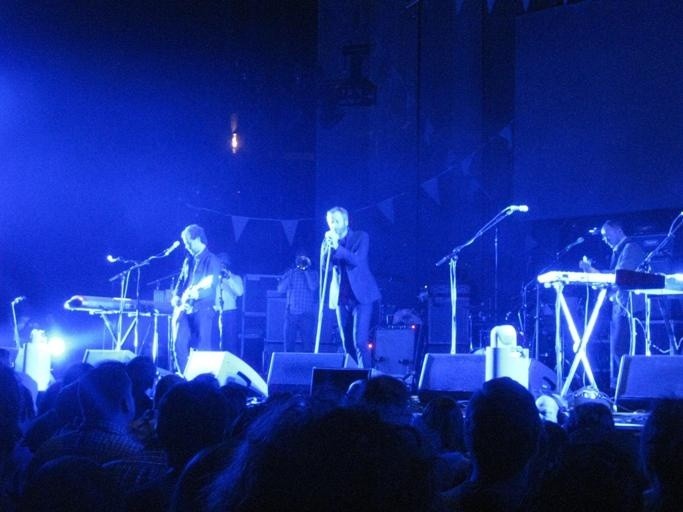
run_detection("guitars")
[172,275,213,324]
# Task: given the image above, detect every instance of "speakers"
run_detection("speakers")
[81,349,138,370]
[182,350,268,399]
[267,352,359,399]
[415,354,488,401]
[511,358,575,401]
[614,354,683,410]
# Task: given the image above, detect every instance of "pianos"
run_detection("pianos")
[537,271,683,289]
[64,296,172,316]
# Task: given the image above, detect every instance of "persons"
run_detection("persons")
[275,256,321,354]
[318,206,381,370]
[169,223,220,378]
[577,216,653,392]
[212,250,244,356]
[12,323,56,392]
[1,342,683,512]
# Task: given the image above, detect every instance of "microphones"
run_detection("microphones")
[12,296,28,304]
[575,224,599,244]
[164,240,181,255]
[509,205,528,213]
[106,254,120,262]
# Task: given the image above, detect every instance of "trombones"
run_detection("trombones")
[277,256,312,281]
